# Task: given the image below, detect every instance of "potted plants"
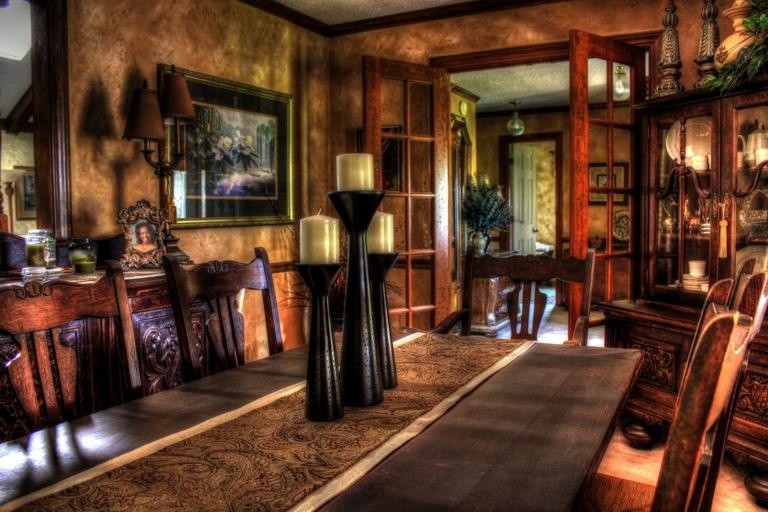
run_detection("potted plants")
[460,174,513,257]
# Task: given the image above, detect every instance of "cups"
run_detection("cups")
[754,148,768,168]
[691,156,709,172]
[689,260,706,277]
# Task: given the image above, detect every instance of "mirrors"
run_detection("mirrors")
[1,0,71,242]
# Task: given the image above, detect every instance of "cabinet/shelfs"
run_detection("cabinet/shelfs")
[628,83,766,303]
[599,297,768,512]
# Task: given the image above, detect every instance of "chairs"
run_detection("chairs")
[121,271,224,397]
[576,257,766,512]
[428,244,596,345]
[0,260,142,436]
[167,249,284,385]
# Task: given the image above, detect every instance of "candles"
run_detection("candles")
[365,213,395,253]
[300,208,340,264]
[337,152,373,190]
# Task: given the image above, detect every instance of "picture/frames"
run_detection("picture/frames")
[351,125,401,194]
[158,61,298,227]
[117,198,171,269]
[586,163,627,206]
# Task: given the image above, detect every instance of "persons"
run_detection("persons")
[133,222,157,251]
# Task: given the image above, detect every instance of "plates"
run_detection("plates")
[681,274,707,289]
[665,116,716,170]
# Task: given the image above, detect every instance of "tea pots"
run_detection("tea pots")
[738,123,768,169]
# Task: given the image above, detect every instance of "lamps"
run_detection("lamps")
[121,72,197,266]
[612,63,631,102]
[506,100,525,137]
